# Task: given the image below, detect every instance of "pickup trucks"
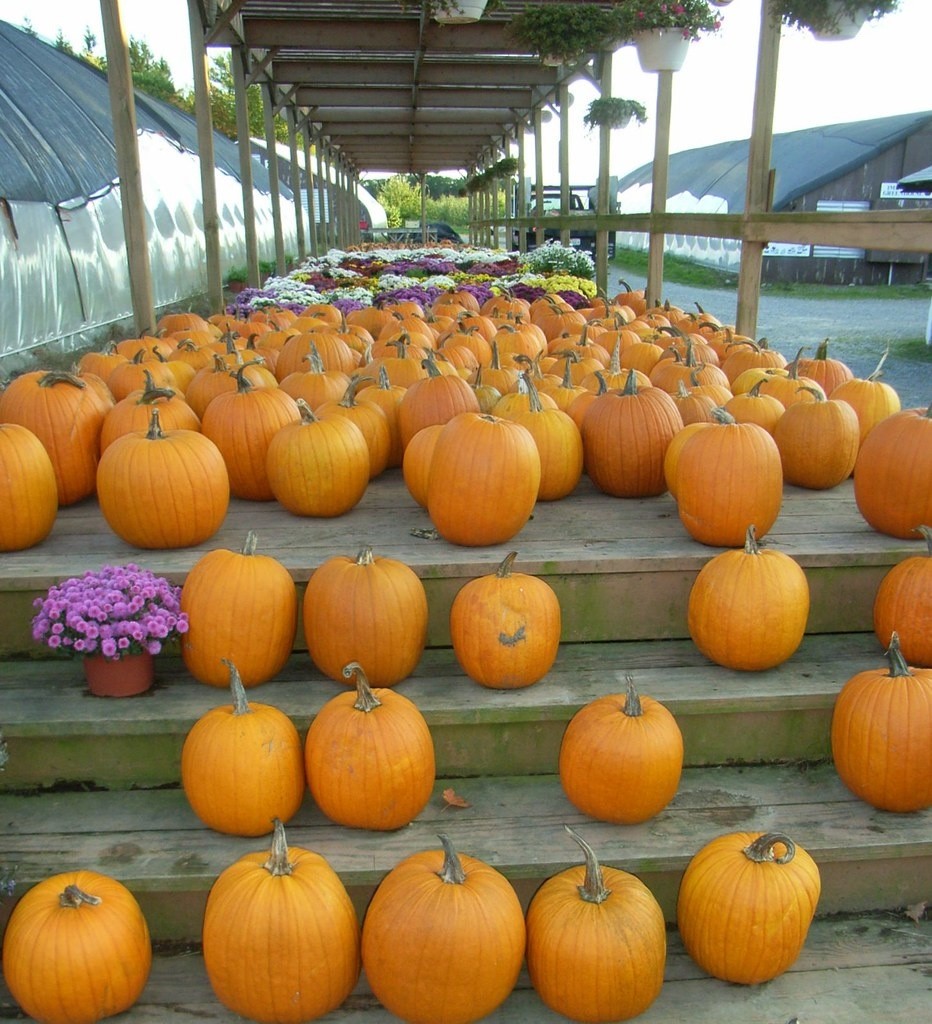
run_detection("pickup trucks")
[511,194,616,261]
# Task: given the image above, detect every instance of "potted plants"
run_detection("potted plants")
[227,266,241,292]
[460,156,518,196]
[768,0,899,43]
[583,97,648,130]
[241,269,248,290]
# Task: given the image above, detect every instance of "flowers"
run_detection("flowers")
[31,563,190,660]
[226,238,598,319]
[628,0,726,41]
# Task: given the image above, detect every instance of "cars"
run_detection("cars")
[414,223,463,244]
[491,226,507,235]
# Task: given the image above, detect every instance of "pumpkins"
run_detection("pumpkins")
[179,526,932,1024]
[0,280,932,551]
[2,870,151,1024]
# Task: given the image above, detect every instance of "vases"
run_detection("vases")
[635,26,690,72]
[81,652,154,697]
[427,0,487,25]
